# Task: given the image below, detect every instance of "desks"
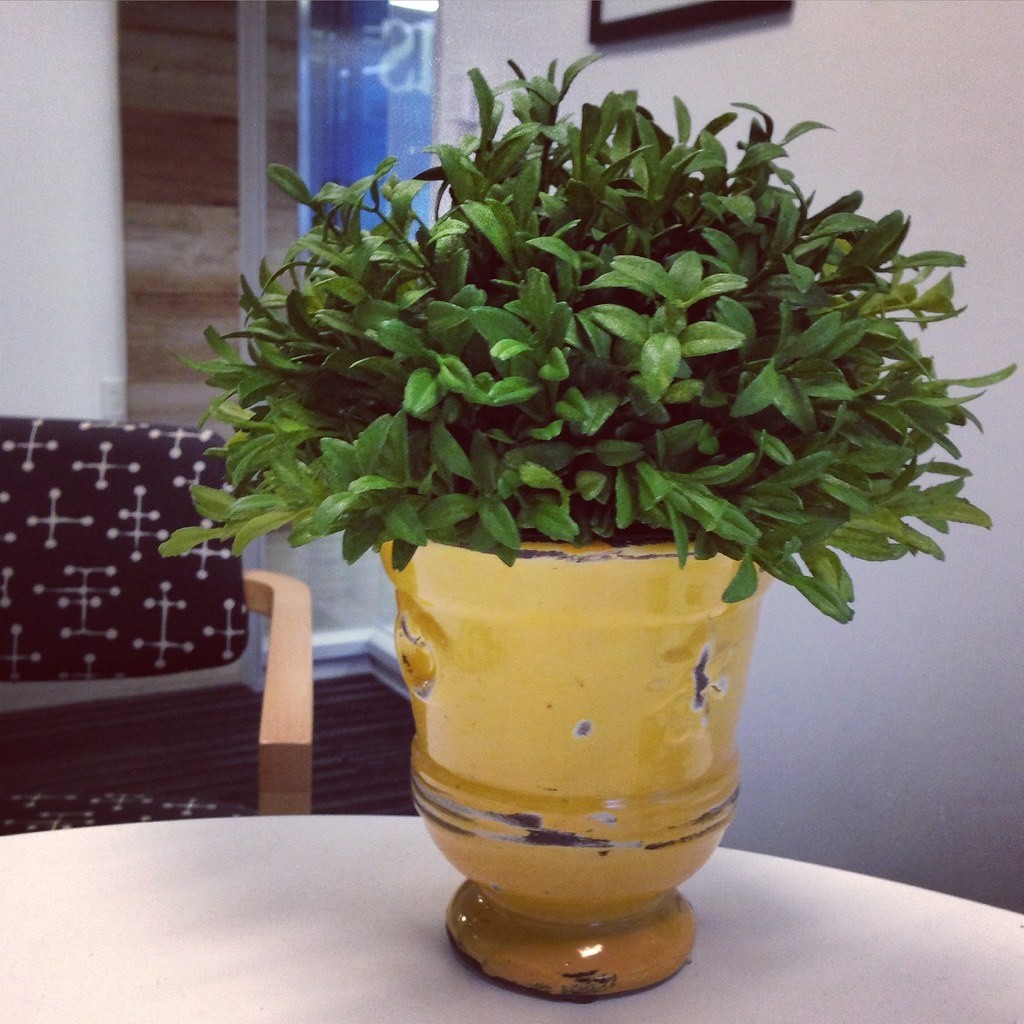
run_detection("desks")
[0,814,1024,1024]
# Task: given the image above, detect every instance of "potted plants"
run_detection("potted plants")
[161,40,1017,1005]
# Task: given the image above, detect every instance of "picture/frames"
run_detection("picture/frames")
[588,0,794,44]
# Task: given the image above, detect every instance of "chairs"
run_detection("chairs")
[0,417,313,835]
